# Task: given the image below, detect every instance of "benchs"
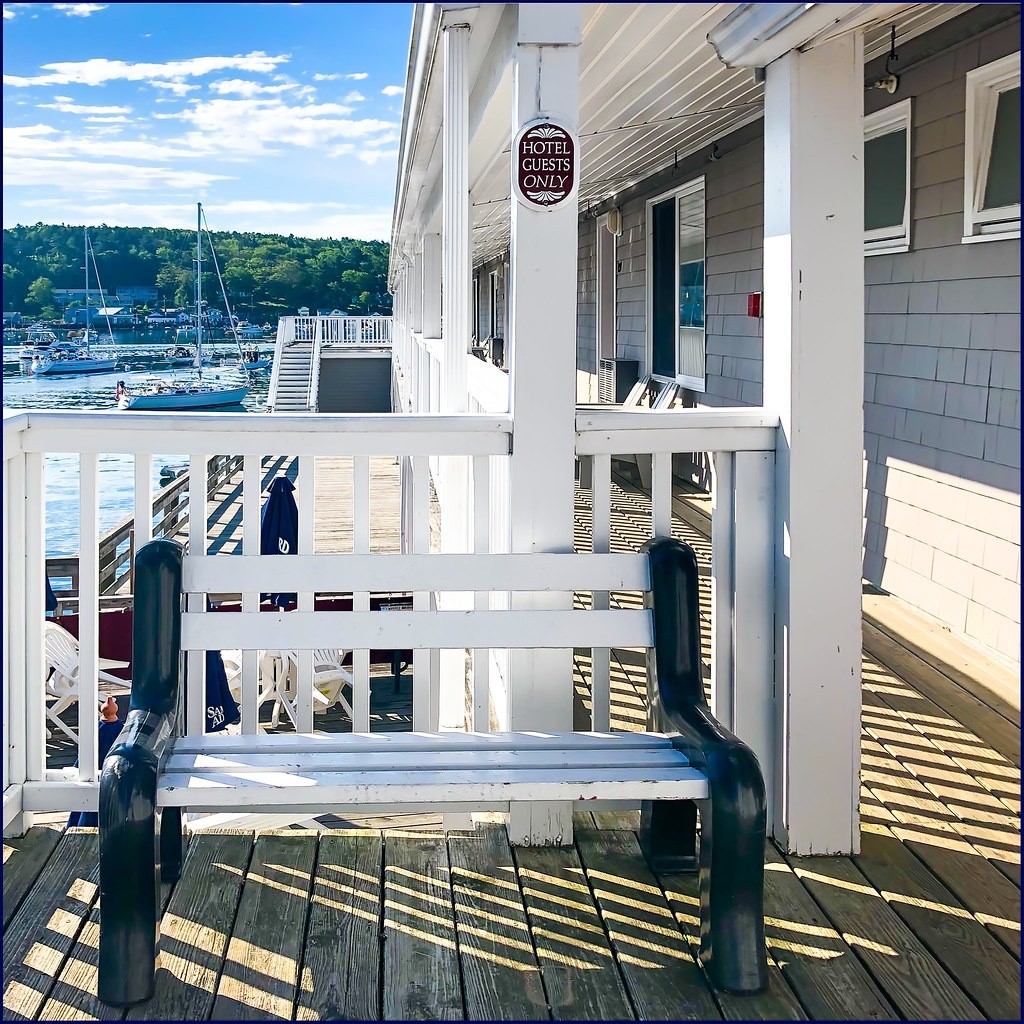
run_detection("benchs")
[95,537,766,1008]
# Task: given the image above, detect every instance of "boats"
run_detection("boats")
[233,319,276,343]
[167,325,215,364]
[216,351,269,374]
[67,328,100,344]
[22,331,59,347]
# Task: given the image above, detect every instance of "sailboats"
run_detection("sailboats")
[29,227,120,376]
[118,202,256,412]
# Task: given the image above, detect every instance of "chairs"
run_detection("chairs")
[575,374,680,490]
[44,620,131,744]
[221,609,372,729]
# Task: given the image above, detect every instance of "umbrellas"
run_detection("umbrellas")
[67,696,124,827]
[184,593,241,737]
[264,475,298,713]
